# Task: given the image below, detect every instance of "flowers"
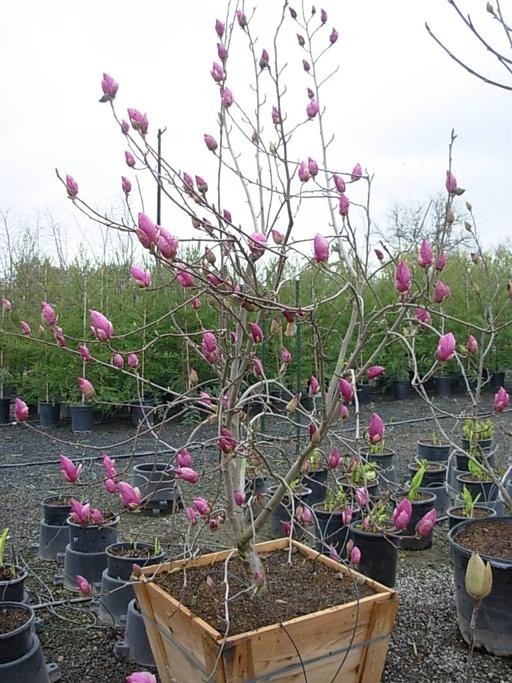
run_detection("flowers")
[0,0,512,683]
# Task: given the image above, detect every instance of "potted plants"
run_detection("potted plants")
[0,182,512,683]
